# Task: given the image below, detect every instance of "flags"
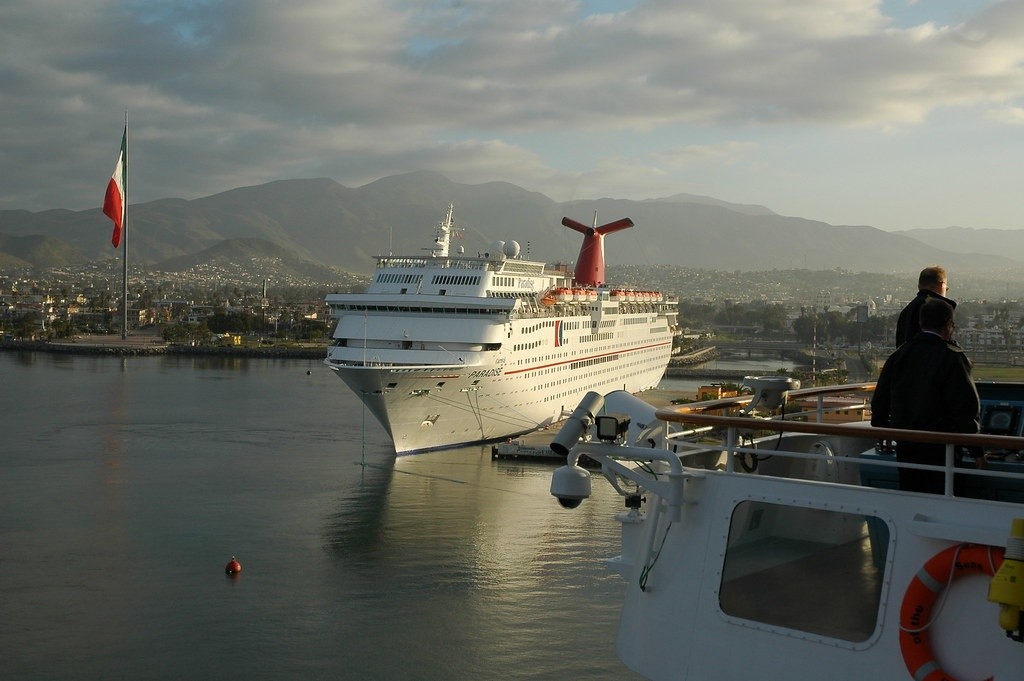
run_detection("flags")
[102,126,126,249]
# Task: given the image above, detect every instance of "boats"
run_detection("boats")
[489,357,1024,681]
[542,287,663,307]
[324,199,682,459]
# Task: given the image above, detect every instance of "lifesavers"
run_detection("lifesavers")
[898,543,1005,681]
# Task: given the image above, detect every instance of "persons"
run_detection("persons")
[870,298,981,497]
[894,266,957,350]
